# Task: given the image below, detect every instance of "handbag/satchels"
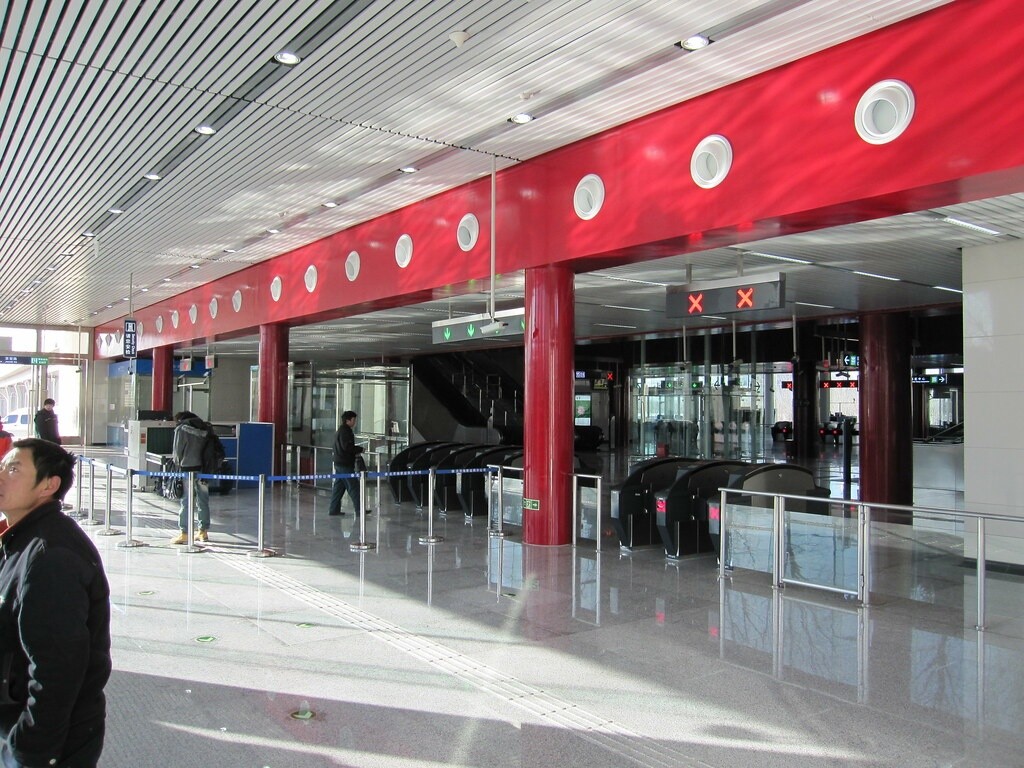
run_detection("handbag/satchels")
[162,458,183,499]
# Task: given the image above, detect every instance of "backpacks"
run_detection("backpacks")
[201,430,225,473]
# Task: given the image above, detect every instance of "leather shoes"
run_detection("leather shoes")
[329,511,345,515]
[356,510,372,516]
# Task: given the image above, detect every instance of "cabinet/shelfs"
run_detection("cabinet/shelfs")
[209,421,276,489]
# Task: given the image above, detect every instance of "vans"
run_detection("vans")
[0,408,63,443]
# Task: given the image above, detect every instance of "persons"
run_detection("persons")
[172,411,210,544]
[1,439,112,768]
[34,398,61,446]
[329,411,374,515]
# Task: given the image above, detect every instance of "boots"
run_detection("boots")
[171,533,188,544]
[194,528,208,542]
